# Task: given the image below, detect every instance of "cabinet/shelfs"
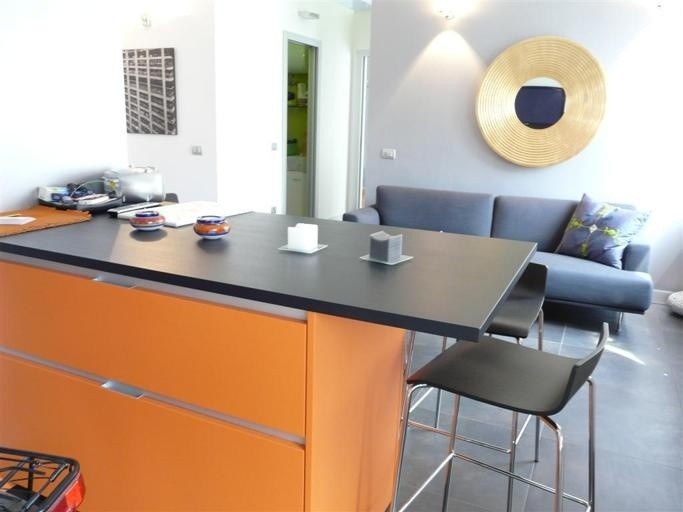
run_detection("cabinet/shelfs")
[0,200,538,512]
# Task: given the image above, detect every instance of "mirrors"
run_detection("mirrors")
[473,35,608,169]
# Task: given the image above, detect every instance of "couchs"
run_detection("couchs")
[342,186,653,336]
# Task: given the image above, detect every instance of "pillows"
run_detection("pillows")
[555,192,650,270]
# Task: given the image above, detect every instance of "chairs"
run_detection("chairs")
[407,263,550,454]
[392,322,610,509]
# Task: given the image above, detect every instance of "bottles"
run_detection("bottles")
[131,210,165,233]
[193,215,231,240]
